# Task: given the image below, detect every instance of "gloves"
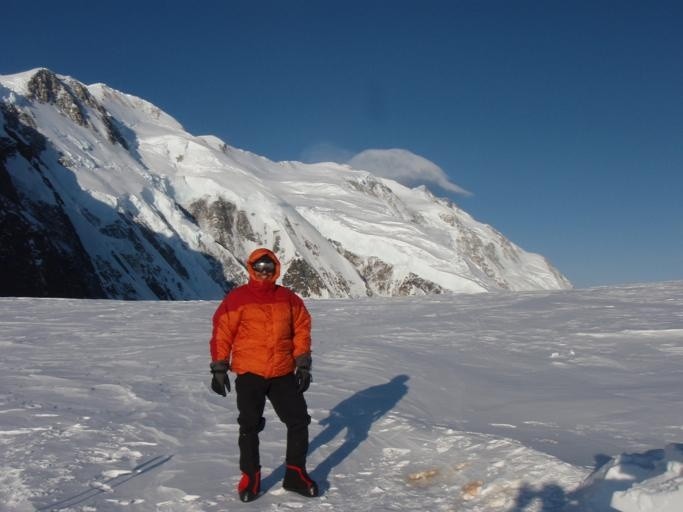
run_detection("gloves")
[209,360,231,397]
[295,352,311,393]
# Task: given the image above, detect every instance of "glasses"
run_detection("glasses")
[253,261,275,273]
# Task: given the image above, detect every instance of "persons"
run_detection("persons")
[209,248,319,502]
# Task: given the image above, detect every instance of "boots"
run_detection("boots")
[237,465,261,502]
[283,465,318,497]
